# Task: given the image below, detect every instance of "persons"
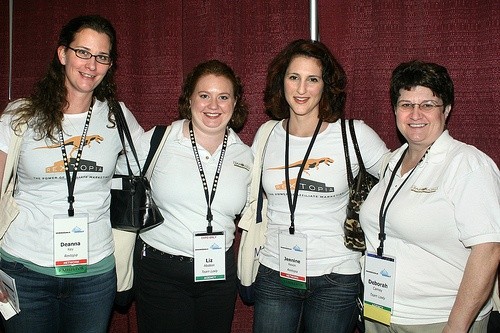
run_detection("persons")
[115,61,254,333]
[358,61,500,333]
[235,40,393,333]
[0,17,150,333]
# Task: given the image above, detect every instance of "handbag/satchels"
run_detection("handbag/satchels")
[109,102,164,234]
[0,192,20,241]
[341,117,381,252]
[237,198,268,306]
[112,228,137,315]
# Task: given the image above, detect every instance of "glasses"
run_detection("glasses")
[68,46,111,65]
